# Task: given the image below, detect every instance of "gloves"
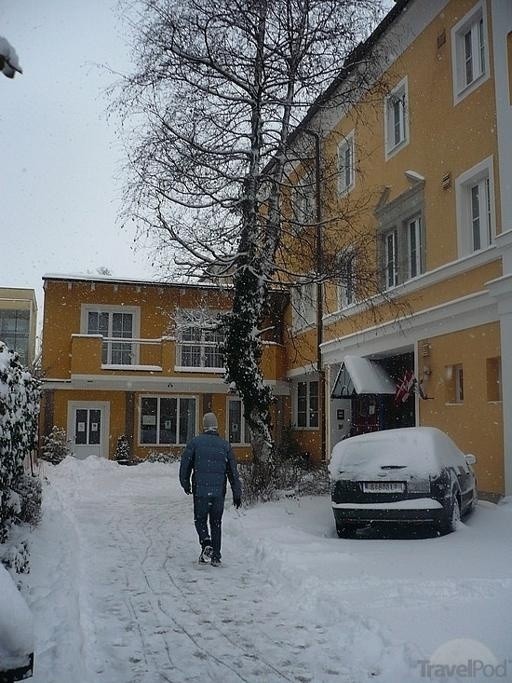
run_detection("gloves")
[185,488,192,495]
[233,496,242,509]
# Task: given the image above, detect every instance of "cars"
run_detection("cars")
[0,561,34,682]
[330,427,478,538]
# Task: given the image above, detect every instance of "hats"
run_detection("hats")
[203,412,218,429]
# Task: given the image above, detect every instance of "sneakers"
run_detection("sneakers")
[211,556,222,567]
[199,540,214,563]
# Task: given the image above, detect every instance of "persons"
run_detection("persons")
[177,412,242,568]
